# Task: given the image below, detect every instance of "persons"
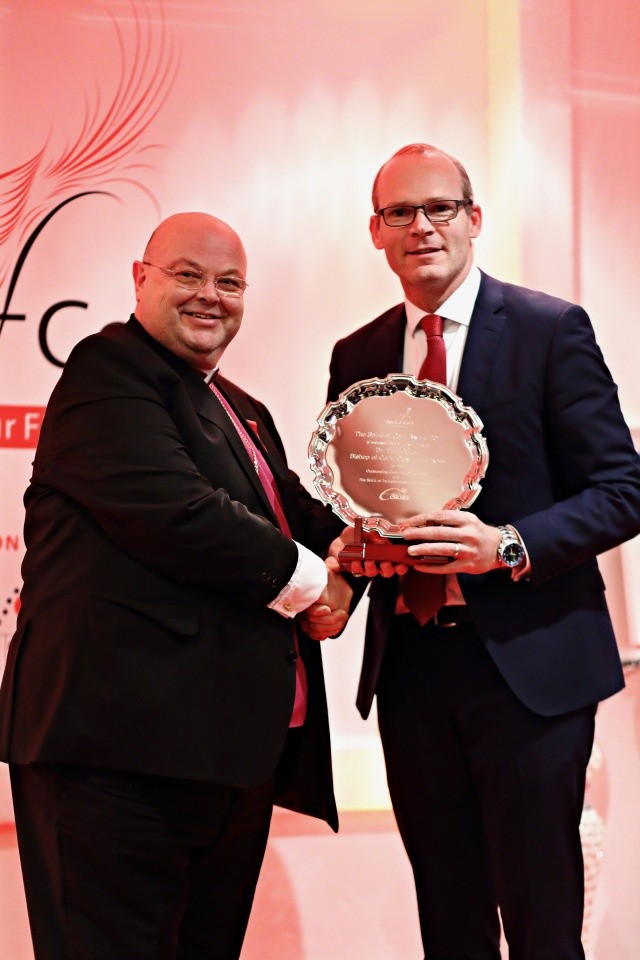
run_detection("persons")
[300,141,640,960]
[0,207,357,959]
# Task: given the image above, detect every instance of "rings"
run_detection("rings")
[453,544,460,558]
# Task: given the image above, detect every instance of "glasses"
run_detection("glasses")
[143,261,249,298]
[378,199,473,227]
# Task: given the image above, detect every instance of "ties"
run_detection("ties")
[402,313,447,626]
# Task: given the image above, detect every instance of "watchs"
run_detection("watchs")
[497,525,525,569]
